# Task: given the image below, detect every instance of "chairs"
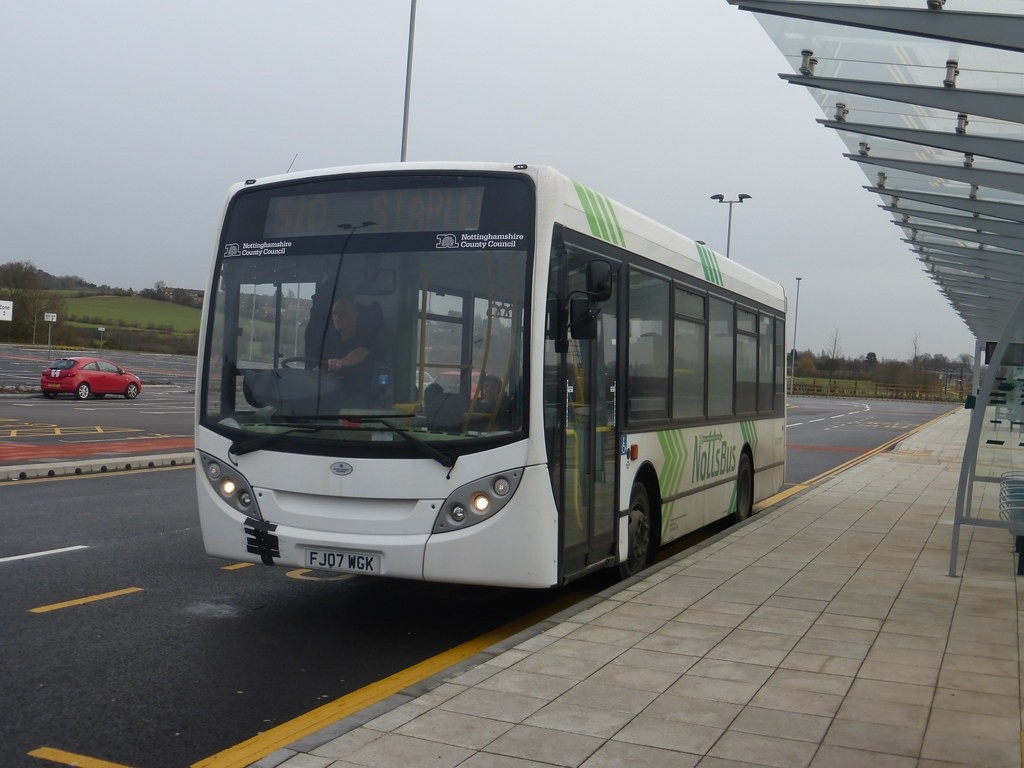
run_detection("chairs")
[998,470,1024,536]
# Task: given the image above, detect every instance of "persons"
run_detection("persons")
[311,298,374,408]
[472,375,508,413]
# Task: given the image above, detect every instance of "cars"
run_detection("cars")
[41,357,142,400]
[414,367,433,391]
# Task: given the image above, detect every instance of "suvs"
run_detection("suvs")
[431,371,484,400]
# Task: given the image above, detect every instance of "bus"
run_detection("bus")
[193,163,789,592]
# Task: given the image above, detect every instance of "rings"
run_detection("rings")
[336,364,338,365]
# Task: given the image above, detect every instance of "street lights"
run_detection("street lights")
[709,193,753,258]
[790,277,803,394]
